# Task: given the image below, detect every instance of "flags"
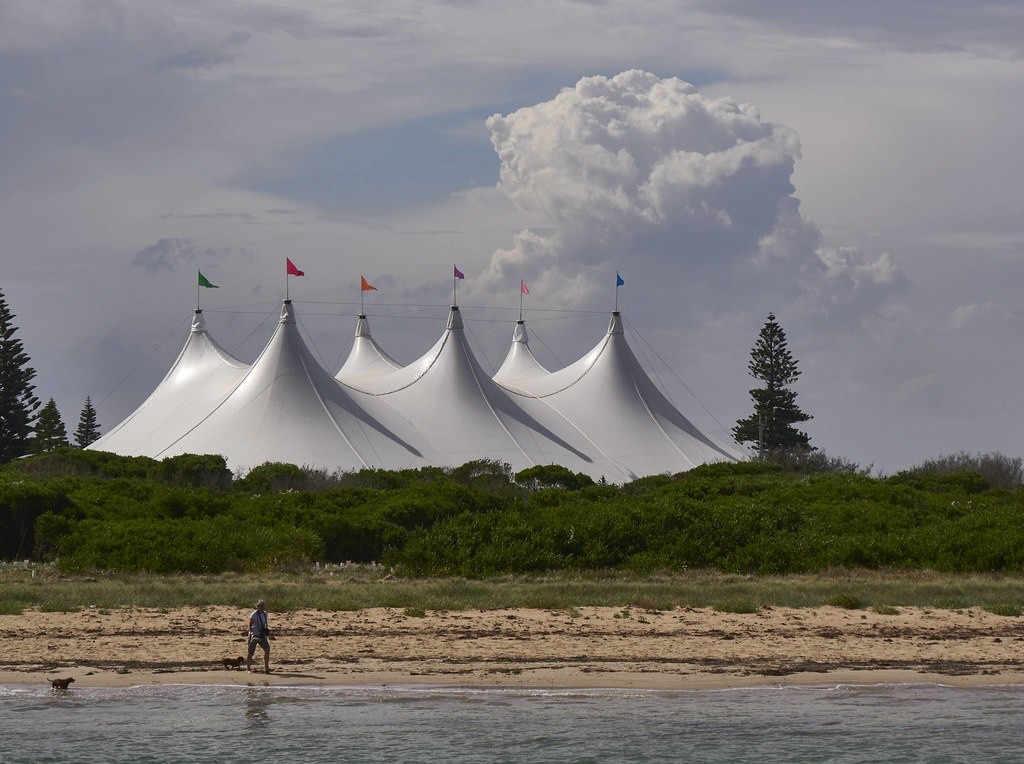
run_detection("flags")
[197,271,219,288]
[361,276,378,291]
[454,265,464,279]
[287,259,304,276]
[520,280,529,294]
[616,273,624,286]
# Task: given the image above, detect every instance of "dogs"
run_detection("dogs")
[47,677,75,689]
[222,657,245,670]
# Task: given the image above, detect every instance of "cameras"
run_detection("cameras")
[264,628,270,636]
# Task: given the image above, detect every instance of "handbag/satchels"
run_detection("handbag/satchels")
[264,628,270,637]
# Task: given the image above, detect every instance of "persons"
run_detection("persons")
[247,599,275,673]
